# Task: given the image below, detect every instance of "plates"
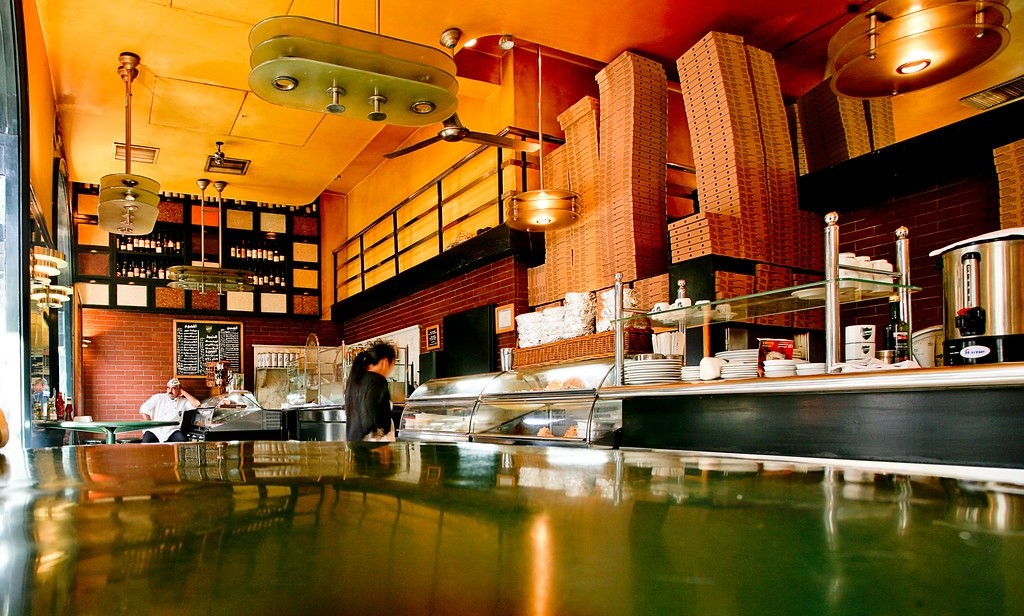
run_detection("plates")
[647,311,738,327]
[763,359,827,377]
[790,287,859,303]
[623,348,758,386]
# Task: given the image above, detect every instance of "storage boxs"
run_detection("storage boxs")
[527,31,896,330]
[994,139,1024,229]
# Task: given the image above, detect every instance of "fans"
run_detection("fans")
[382,28,541,159]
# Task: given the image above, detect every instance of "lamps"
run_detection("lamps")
[28,225,74,311]
[82,337,92,347]
[166,179,255,295]
[505,45,582,234]
[829,0,1012,99]
[99,54,160,237]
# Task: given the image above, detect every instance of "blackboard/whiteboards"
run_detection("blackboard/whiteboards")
[173,319,244,378]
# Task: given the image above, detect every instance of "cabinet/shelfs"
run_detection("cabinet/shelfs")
[114,221,188,287]
[222,229,288,295]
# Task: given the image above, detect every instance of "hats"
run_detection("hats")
[168,378,181,385]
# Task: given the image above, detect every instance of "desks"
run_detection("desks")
[35,422,180,444]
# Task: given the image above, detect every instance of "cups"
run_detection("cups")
[700,357,720,381]
[651,298,732,314]
[836,253,893,296]
[258,352,300,368]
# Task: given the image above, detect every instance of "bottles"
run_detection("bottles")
[883,303,909,365]
[84,183,317,287]
[32,392,73,421]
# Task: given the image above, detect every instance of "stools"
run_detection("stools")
[116,437,142,444]
[81,439,105,444]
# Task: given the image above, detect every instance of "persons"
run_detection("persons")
[344,343,397,441]
[140,378,201,443]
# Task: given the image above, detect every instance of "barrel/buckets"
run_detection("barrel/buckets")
[941,236,1024,369]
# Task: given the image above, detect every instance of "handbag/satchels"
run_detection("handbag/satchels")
[362,401,396,441]
